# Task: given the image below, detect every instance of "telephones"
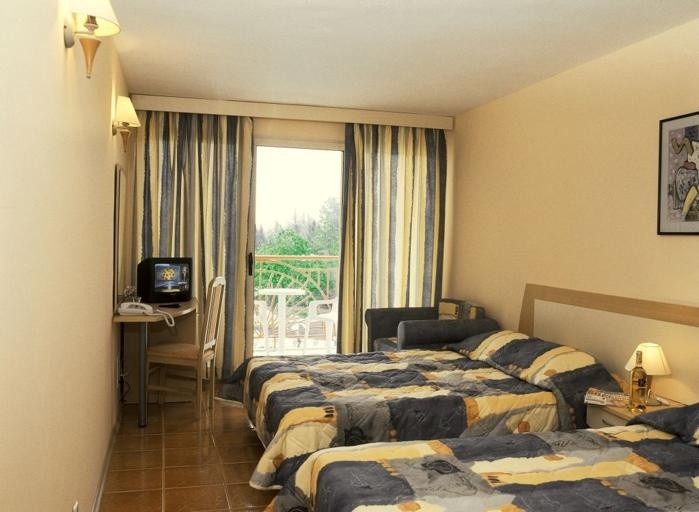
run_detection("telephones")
[118,302,153,316]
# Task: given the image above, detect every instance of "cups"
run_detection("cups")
[133,296,141,304]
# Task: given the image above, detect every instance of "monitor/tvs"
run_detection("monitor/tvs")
[137,256,193,303]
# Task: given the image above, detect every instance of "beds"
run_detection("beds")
[264,404,699,512]
[218,284,699,491]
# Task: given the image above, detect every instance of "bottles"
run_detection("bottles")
[628,350,649,416]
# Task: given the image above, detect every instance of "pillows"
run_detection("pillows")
[626,403,699,445]
[443,329,622,430]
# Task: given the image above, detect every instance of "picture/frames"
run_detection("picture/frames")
[658,111,699,235]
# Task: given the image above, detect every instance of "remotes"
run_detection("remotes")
[159,304,180,308]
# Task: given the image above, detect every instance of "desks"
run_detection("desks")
[259,288,305,355]
[113,298,197,427]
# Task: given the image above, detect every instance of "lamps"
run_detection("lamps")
[624,343,671,398]
[112,96,141,152]
[64,0,121,78]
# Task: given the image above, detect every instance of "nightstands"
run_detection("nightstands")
[586,394,688,428]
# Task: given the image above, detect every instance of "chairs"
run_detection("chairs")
[305,296,339,352]
[254,300,270,355]
[145,277,226,424]
[365,299,498,352]
